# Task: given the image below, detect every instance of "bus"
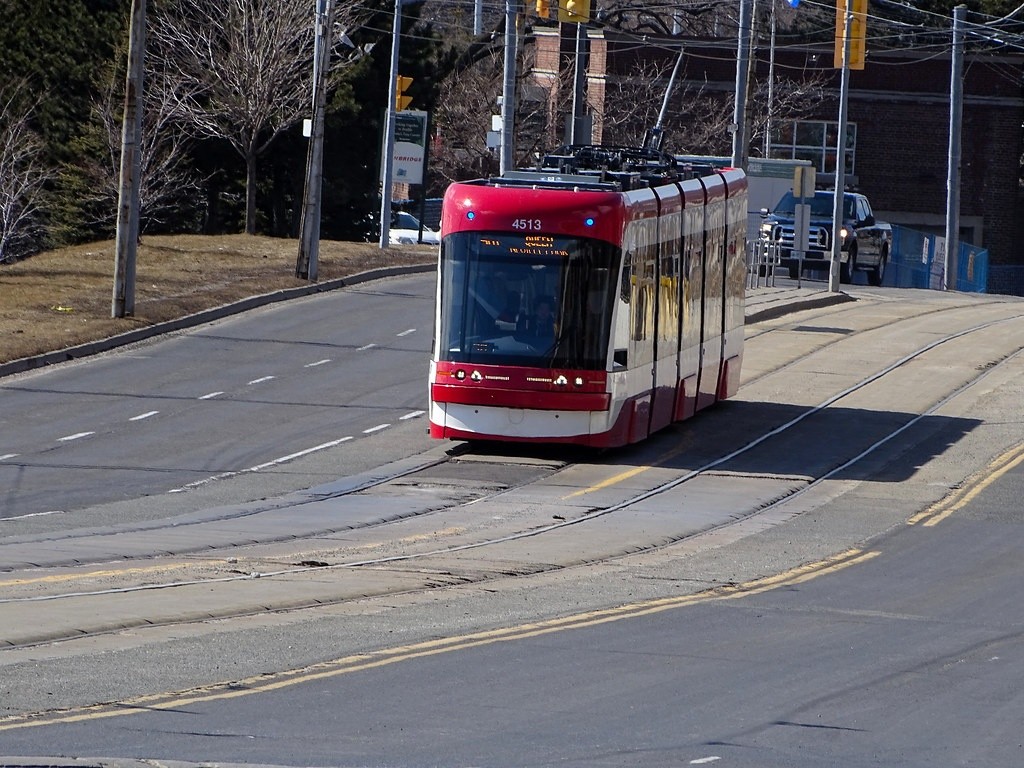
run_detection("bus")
[427,49,749,449]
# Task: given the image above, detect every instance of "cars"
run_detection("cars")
[360,211,440,246]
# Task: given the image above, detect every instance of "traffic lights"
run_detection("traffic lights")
[395,75,414,112]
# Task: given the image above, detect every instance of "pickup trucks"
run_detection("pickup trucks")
[758,188,893,286]
